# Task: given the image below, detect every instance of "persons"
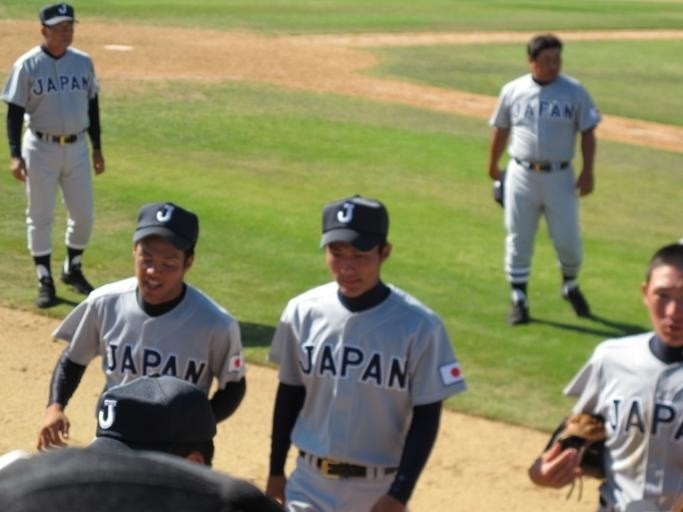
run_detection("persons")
[0,2,105,309]
[266,194,467,511]
[95,370,216,467]
[36,201,247,449]
[487,35,602,325]
[527,244,683,511]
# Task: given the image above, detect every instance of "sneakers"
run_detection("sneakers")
[507,301,530,326]
[559,285,590,319]
[35,282,57,308]
[62,268,94,296]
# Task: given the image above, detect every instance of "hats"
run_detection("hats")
[492,170,507,207]
[129,199,200,253]
[316,195,394,250]
[85,373,220,455]
[296,448,403,481]
[36,1,79,28]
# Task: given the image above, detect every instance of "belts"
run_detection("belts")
[26,128,88,145]
[514,157,572,172]
